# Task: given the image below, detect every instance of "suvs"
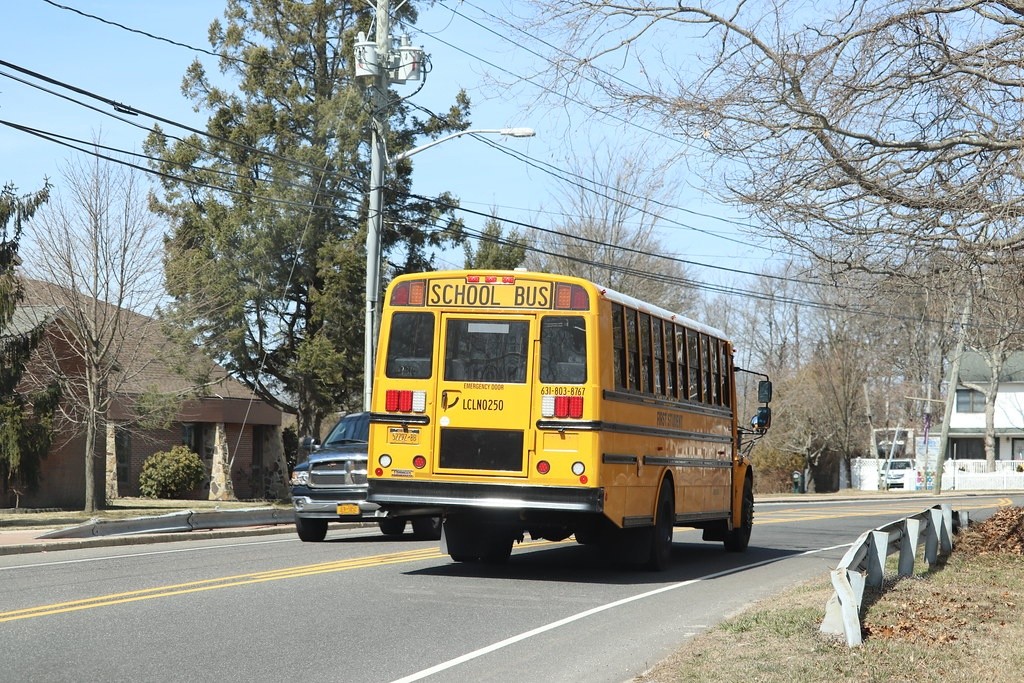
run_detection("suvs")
[288,411,445,542]
[879,458,915,493]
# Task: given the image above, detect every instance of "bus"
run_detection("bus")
[366,267,772,574]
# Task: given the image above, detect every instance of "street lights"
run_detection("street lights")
[363,127,537,412]
